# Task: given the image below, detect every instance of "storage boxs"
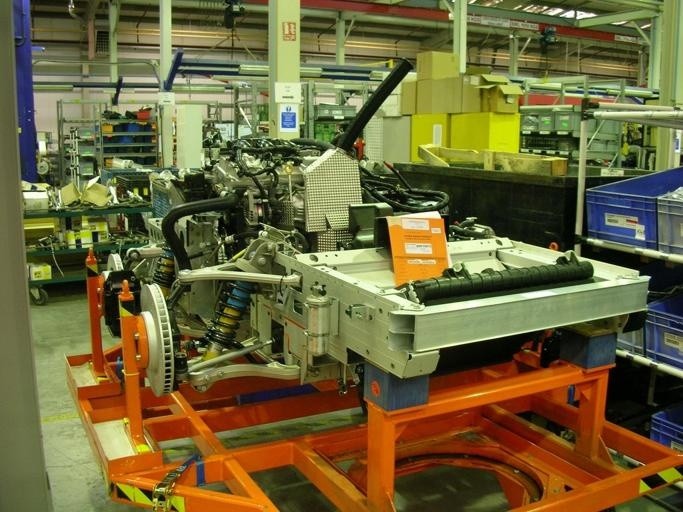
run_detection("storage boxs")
[66,216,108,249]
[23,192,49,212]
[28,262,51,281]
[586,167,683,250]
[650,410,683,452]
[644,288,682,370]
[400,50,596,165]
[658,191,683,254]
[617,294,669,356]
